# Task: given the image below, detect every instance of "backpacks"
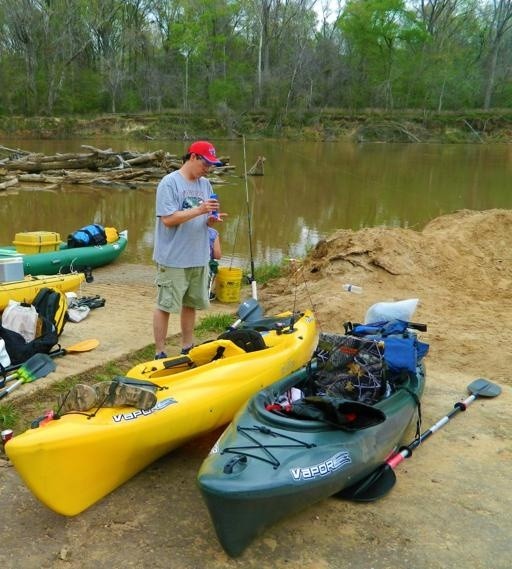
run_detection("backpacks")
[31,287,69,336]
[68,224,106,246]
[1,318,60,363]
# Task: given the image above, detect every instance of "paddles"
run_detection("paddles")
[329,378,501,502]
[225,298,262,332]
[0,338,99,400]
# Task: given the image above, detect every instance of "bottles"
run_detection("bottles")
[207,194,218,220]
[342,283,362,294]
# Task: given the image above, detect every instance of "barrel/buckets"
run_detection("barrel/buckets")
[215,265,243,303]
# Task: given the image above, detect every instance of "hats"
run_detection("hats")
[188,141,223,164]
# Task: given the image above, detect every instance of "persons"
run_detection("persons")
[153,141,228,360]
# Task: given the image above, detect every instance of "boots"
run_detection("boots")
[58,377,157,411]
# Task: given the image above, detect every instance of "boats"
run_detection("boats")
[0,229,129,276]
[194,358,427,559]
[0,272,86,313]
[4,307,318,518]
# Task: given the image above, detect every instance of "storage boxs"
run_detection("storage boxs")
[10,231,62,253]
[0,257,24,283]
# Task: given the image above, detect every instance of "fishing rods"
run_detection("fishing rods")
[242,133,257,302]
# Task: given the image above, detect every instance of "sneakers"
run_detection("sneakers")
[181,344,194,354]
[154,352,167,359]
